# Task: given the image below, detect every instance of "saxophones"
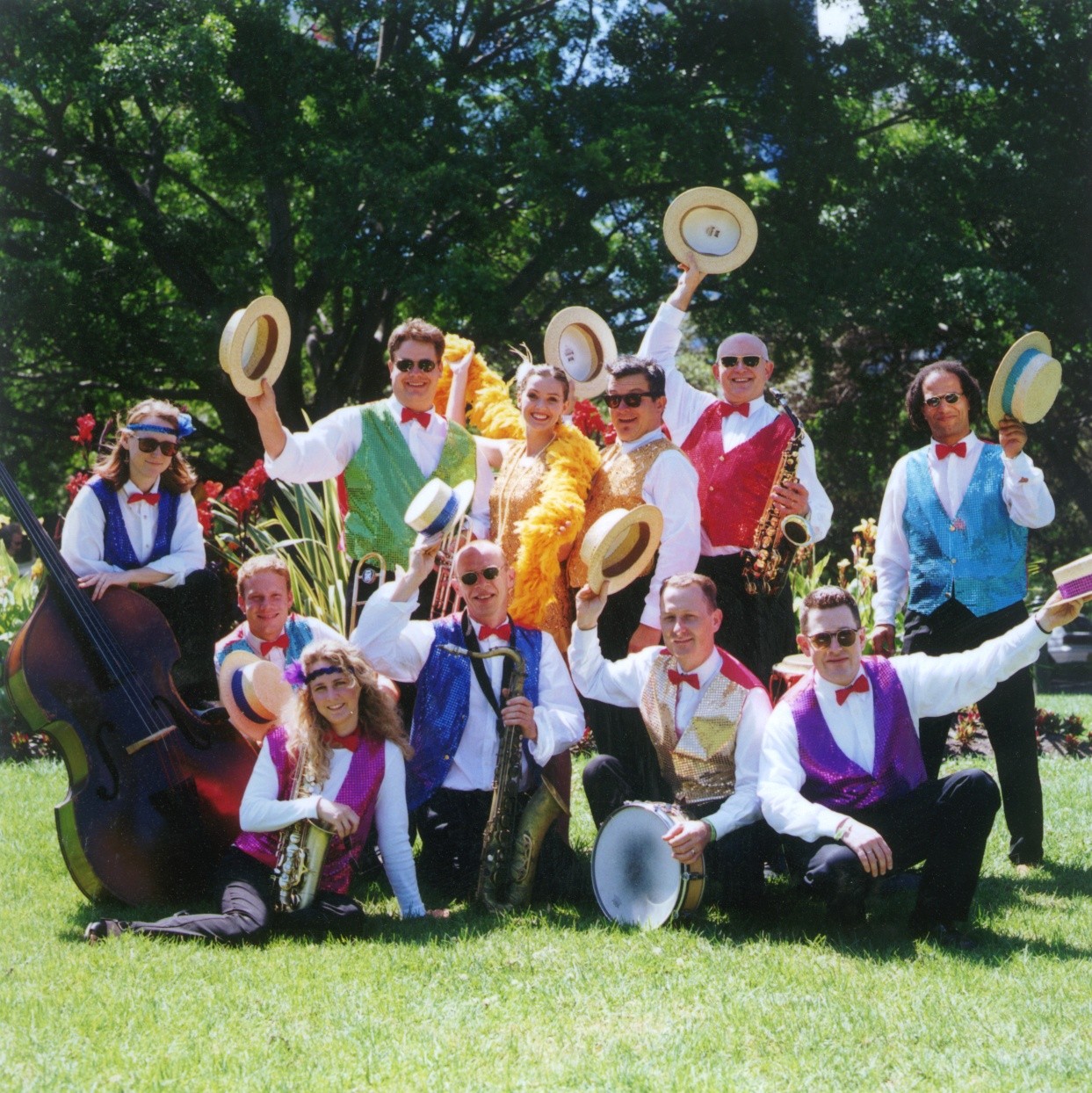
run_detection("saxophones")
[268,729,339,917]
[738,387,814,603]
[436,641,572,919]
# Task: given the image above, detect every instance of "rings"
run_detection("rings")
[691,849,696,856]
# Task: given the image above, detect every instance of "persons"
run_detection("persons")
[243,317,497,846]
[348,527,586,900]
[756,585,1091,947]
[84,640,454,943]
[566,571,774,913]
[445,355,700,778]
[872,359,1056,869]
[54,396,350,744]
[635,251,835,701]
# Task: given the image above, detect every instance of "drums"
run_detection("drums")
[589,798,709,935]
[768,653,814,709]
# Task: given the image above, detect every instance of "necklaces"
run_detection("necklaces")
[495,432,558,542]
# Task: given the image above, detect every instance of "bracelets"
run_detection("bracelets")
[834,818,854,841]
[1034,616,1053,635]
[703,819,716,842]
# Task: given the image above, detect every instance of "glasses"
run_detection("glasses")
[717,355,769,368]
[603,391,657,409]
[807,628,861,650]
[392,359,441,373]
[128,432,179,456]
[457,562,506,586]
[922,392,966,409]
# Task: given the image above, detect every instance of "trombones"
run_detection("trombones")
[348,550,388,642]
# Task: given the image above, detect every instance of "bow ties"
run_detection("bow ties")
[127,492,161,506]
[324,727,361,753]
[936,442,966,459]
[716,402,750,417]
[478,624,511,640]
[835,674,870,706]
[401,407,431,429]
[260,634,290,657]
[667,669,699,690]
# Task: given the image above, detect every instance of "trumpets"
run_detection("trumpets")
[427,513,473,623]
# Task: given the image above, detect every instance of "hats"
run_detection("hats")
[544,306,618,402]
[404,477,474,547]
[219,650,293,742]
[1045,552,1092,609]
[219,295,291,397]
[579,504,662,595]
[987,332,1062,431]
[662,186,758,274]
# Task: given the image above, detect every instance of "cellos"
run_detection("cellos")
[0,458,259,913]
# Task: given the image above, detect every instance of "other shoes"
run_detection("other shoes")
[84,918,108,943]
[910,922,979,951]
[831,914,869,932]
